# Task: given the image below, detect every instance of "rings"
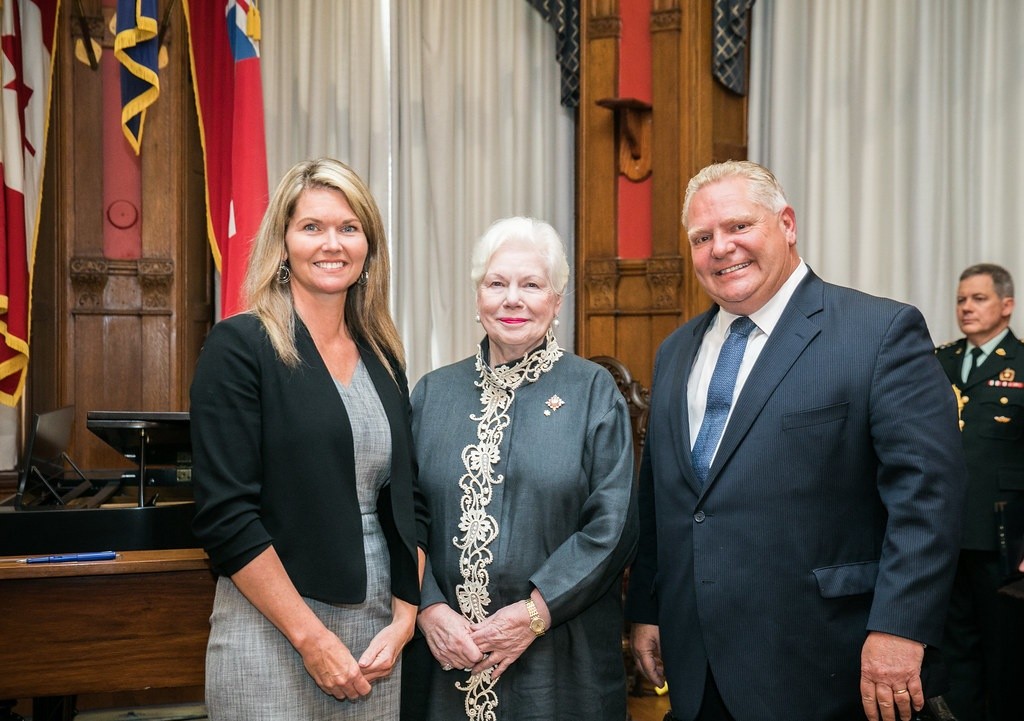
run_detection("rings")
[893,689,908,694]
[441,663,452,671]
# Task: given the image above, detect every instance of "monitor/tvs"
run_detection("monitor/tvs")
[85,408,192,507]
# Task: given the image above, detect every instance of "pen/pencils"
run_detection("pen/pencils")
[17,550,119,564]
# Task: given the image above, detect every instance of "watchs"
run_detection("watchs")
[525,597,546,637]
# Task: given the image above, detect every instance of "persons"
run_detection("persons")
[409,216,634,721]
[623,160,964,721]
[188,157,430,721]
[934,263,1024,585]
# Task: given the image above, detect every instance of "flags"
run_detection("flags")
[183,0,269,320]
[0,0,61,408]
[114,0,160,155]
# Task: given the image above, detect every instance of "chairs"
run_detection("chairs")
[586,355,651,485]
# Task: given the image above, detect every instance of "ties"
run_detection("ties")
[691,317,757,487]
[967,348,983,383]
